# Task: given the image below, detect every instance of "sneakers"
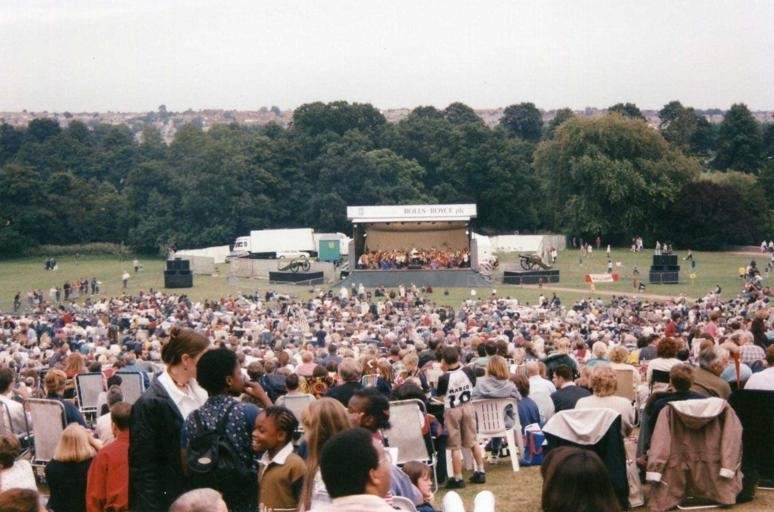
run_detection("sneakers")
[468,470,487,484]
[441,491,464,512]
[444,478,465,488]
[473,490,494,511]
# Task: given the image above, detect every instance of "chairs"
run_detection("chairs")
[471,398,525,472]
[275,394,316,441]
[23,398,68,468]
[612,368,640,427]
[0,400,33,447]
[676,397,727,510]
[74,372,108,428]
[649,369,670,394]
[380,398,438,495]
[113,370,145,405]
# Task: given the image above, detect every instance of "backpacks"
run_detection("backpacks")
[187,399,253,506]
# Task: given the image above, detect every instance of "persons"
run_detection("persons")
[0,230,774,512]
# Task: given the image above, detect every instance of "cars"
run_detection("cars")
[276,248,310,259]
[226,251,254,263]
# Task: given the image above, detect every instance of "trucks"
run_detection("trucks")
[315,232,353,255]
[232,227,316,258]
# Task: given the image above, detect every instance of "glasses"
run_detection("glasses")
[376,449,393,466]
